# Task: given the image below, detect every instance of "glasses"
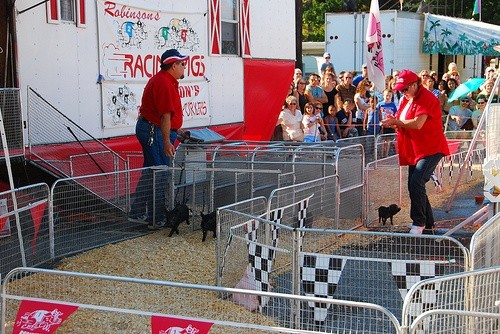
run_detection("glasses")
[345,77,352,79]
[300,83,306,84]
[296,73,302,75]
[315,107,322,110]
[181,63,186,66]
[453,74,458,76]
[365,84,369,86]
[325,57,330,59]
[401,82,414,93]
[478,102,484,104]
[462,100,469,102]
[488,70,494,72]
[290,103,296,105]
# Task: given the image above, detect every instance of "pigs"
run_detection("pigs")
[292,211,313,236]
[162,204,193,237]
[378,204,401,226]
[200,211,222,242]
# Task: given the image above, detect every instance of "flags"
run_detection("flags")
[472,0,481,15]
[366,0,385,93]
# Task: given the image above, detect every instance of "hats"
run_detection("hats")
[160,49,189,65]
[324,52,330,57]
[392,70,421,90]
[451,71,458,74]
[362,64,367,68]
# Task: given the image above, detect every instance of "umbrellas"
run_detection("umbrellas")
[447,78,486,105]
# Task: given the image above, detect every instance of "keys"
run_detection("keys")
[149,125,154,146]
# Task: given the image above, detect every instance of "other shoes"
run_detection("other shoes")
[148,221,165,229]
[407,223,413,228]
[127,215,151,225]
[409,226,425,234]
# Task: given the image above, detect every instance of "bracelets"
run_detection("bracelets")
[331,133,334,136]
[396,120,399,127]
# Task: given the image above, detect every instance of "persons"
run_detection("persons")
[320,52,335,73]
[126,49,189,229]
[419,62,500,148]
[275,65,404,160]
[382,68,451,236]
